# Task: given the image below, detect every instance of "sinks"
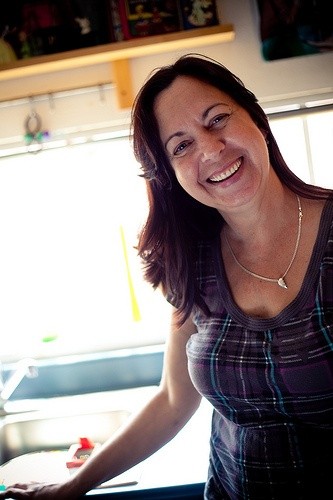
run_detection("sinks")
[0,407,137,497]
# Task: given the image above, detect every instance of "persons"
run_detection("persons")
[0,56,332,499]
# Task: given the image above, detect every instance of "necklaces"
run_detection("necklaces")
[222,192,302,289]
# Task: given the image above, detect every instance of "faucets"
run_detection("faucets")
[22,328,57,365]
